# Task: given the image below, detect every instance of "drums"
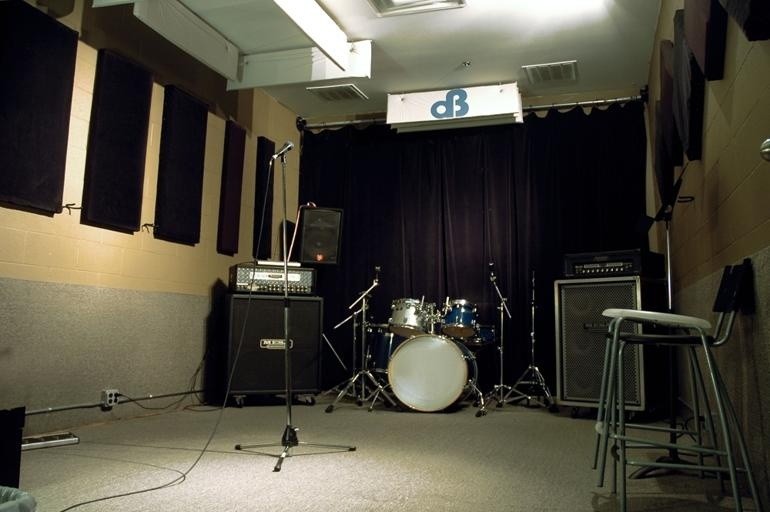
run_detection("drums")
[388,334,478,412]
[461,324,494,346]
[388,298,431,337]
[364,322,407,374]
[441,299,479,337]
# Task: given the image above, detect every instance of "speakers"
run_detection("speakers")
[227,291,324,407]
[298,205,344,265]
[551,275,666,413]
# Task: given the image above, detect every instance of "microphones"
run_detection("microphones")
[488,260,496,283]
[271,139,296,164]
[373,264,381,284]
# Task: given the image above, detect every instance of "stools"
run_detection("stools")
[588,308,745,512]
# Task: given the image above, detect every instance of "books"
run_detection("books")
[257,258,301,268]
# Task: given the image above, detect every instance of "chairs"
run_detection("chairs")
[604,256,764,511]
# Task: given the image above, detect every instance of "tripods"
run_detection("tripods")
[322,323,398,413]
[234,174,358,475]
[481,290,557,413]
